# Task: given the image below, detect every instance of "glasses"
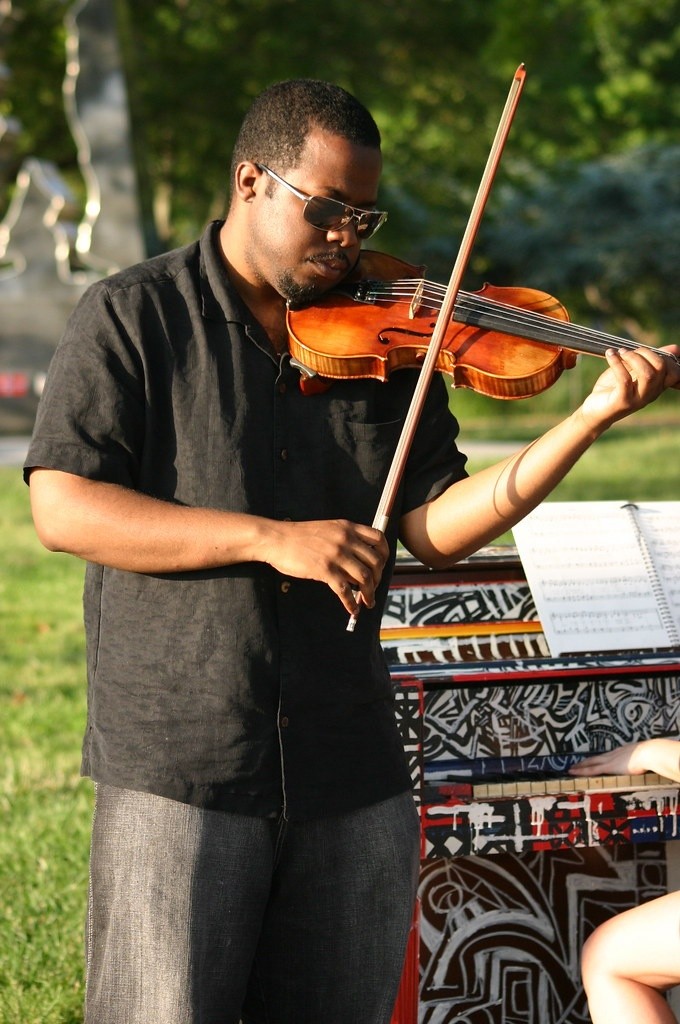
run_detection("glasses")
[255,164,387,241]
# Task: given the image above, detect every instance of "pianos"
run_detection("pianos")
[375,548,680,1023]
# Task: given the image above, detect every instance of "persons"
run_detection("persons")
[24,77,680,1024]
[568,736,680,1024]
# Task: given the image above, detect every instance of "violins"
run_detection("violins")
[284,247,678,405]
